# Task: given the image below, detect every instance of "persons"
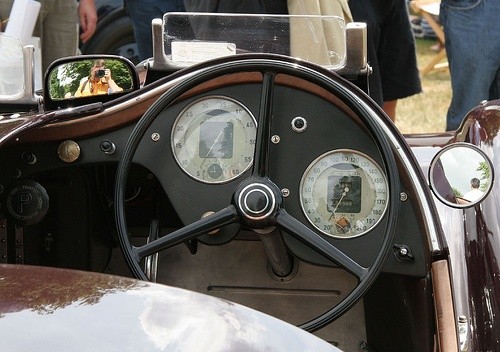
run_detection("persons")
[439,0,499,133]
[125,0,422,128]
[0,1,97,98]
[75,60,123,98]
[462,178,486,202]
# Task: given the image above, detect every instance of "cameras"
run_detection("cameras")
[95,69,106,78]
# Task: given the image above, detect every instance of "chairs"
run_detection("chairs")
[410,0,446,77]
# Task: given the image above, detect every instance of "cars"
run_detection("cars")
[76,1,140,66]
